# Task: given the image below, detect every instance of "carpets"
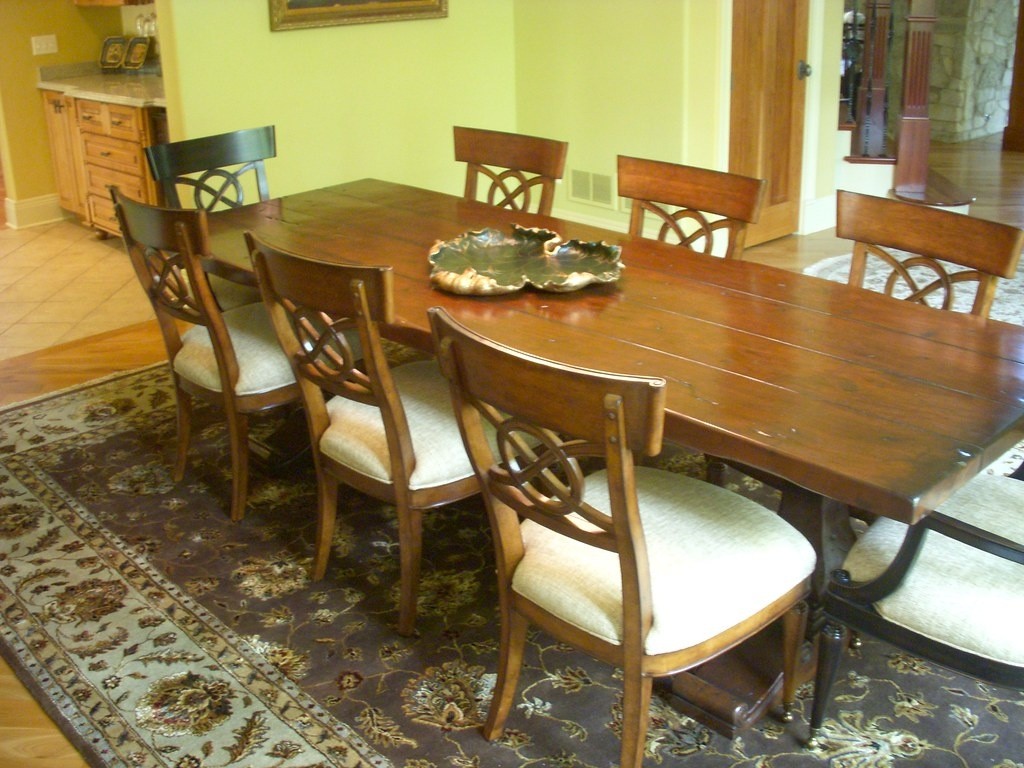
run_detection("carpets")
[804,248,1024,484]
[0,360,1024,768]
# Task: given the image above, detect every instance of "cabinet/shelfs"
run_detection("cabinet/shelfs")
[36,72,170,242]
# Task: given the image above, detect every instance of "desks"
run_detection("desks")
[187,181,1024,768]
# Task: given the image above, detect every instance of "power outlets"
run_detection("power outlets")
[30,34,60,56]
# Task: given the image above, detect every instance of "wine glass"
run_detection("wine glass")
[136,0,157,37]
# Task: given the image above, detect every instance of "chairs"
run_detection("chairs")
[100,121,1024,768]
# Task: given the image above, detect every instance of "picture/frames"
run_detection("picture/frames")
[269,0,448,33]
